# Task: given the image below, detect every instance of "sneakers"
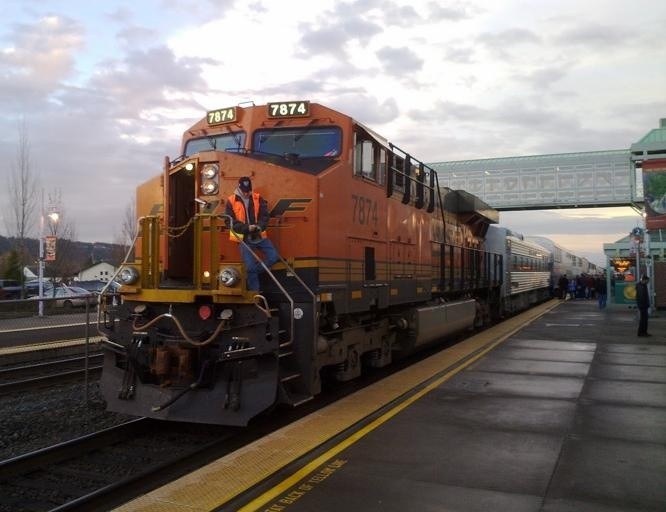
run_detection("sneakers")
[638,332,652,336]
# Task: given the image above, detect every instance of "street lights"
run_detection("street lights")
[39,203,59,317]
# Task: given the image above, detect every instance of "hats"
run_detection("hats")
[238,177,252,192]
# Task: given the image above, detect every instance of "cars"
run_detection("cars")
[0,277,122,309]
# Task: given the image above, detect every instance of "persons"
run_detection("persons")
[225,177,280,290]
[560,273,608,308]
[636,277,651,336]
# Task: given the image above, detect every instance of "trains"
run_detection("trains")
[98,100,606,429]
